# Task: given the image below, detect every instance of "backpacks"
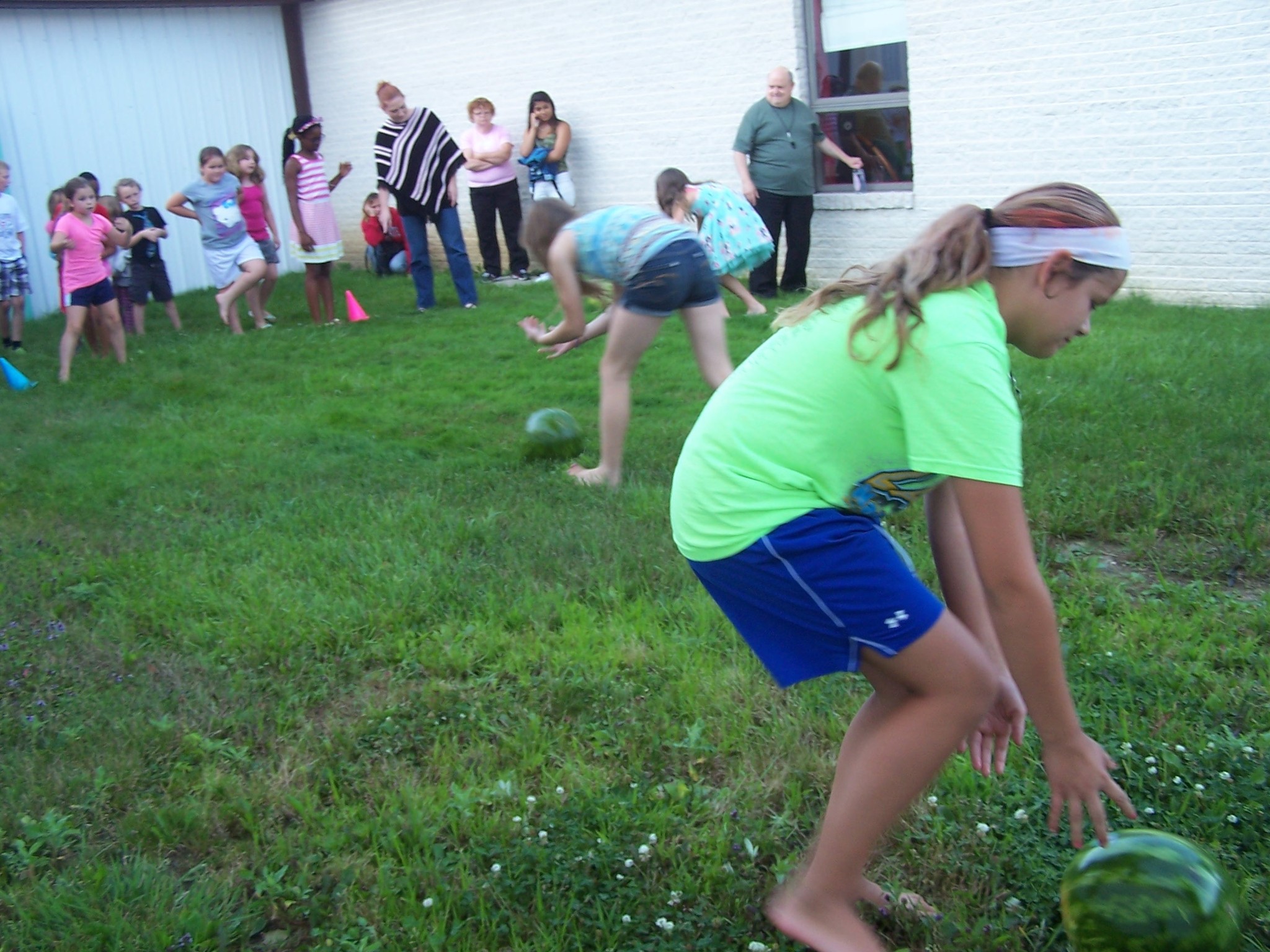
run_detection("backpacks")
[376,240,404,276]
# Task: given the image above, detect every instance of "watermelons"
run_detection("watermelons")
[1061,828,1244,952]
[524,407,585,461]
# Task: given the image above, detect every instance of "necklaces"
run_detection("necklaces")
[770,101,797,150]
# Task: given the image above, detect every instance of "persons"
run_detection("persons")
[463,97,530,282]
[656,168,775,319]
[99,195,133,331]
[49,171,133,383]
[670,181,1138,952]
[820,60,912,182]
[519,92,576,282]
[281,114,353,327]
[114,179,183,335]
[0,161,33,355]
[362,193,411,278]
[376,81,479,315]
[731,67,864,300]
[225,144,281,321]
[517,198,734,489]
[166,146,269,335]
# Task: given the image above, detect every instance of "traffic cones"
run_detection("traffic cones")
[344,289,369,322]
[0,357,39,393]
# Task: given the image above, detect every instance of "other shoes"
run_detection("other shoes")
[479,272,503,284]
[248,309,277,330]
[417,307,427,312]
[317,317,340,328]
[7,346,27,355]
[463,303,476,310]
[511,269,531,280]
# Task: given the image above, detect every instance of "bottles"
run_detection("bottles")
[852,168,867,192]
